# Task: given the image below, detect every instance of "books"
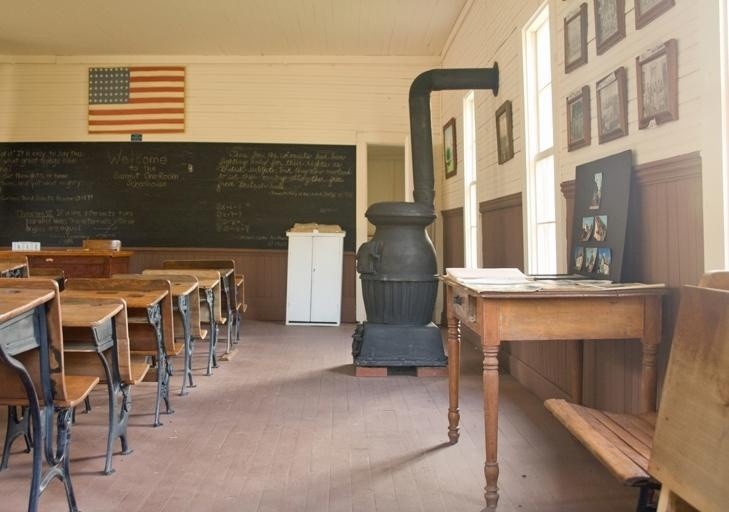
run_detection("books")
[443,264,544,294]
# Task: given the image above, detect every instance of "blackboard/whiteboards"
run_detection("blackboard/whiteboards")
[0,142,356,255]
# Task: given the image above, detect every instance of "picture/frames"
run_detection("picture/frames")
[442,117,457,179]
[494,101,515,166]
[595,66,629,145]
[634,0,676,30]
[564,84,593,150]
[563,3,588,72]
[634,39,679,130]
[593,0,627,58]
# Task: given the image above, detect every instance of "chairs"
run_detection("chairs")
[2,251,248,512]
[544,269,729,511]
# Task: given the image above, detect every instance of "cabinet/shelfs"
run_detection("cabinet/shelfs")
[285,232,347,327]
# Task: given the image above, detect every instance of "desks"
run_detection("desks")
[442,273,667,511]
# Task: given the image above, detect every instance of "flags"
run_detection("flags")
[87,64,186,135]
